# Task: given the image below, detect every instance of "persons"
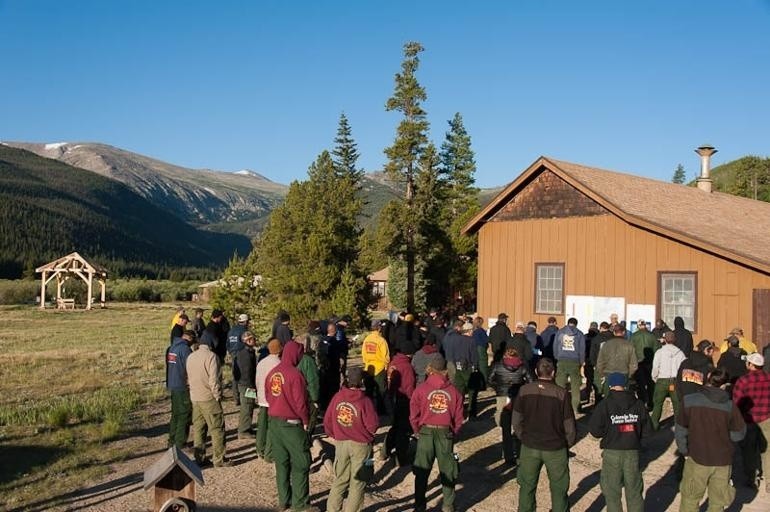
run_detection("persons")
[323,368,379,512]
[408,356,463,512]
[588,373,652,512]
[675,368,746,512]
[512,358,577,512]
[265,340,321,511]
[163,299,770,467]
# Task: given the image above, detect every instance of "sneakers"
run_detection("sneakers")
[238,432,257,439]
[213,458,234,467]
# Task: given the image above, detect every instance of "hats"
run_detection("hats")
[241,331,259,342]
[179,314,192,322]
[371,318,382,327]
[637,318,646,328]
[723,328,744,343]
[608,372,626,386]
[741,352,765,366]
[610,313,618,319]
[238,314,251,322]
[462,313,557,331]
